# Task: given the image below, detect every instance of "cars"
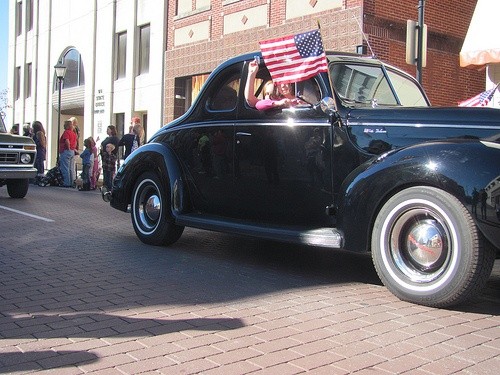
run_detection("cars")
[103,49,500,308]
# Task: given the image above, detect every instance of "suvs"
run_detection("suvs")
[0,113,39,199]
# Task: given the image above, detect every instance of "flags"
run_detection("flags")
[259,29,328,86]
[458,88,496,106]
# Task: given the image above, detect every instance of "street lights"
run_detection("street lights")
[54,60,67,162]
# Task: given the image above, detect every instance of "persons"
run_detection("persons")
[243,56,275,107]
[8,117,145,194]
[255,83,310,110]
